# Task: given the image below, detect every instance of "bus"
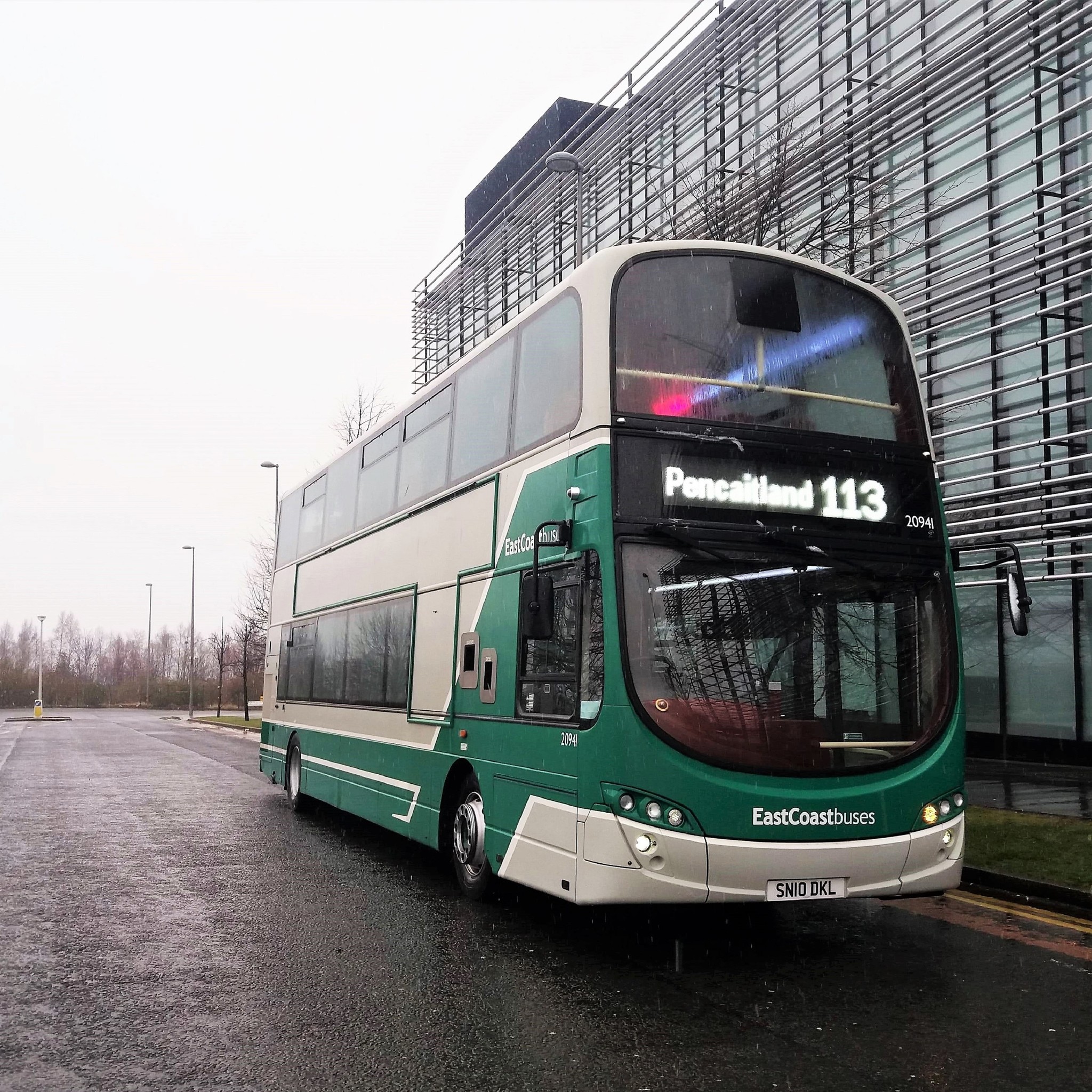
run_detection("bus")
[257,239,1032,916]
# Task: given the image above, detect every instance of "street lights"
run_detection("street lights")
[145,582,152,707]
[182,545,195,717]
[544,151,584,269]
[37,616,47,700]
[261,460,279,528]
